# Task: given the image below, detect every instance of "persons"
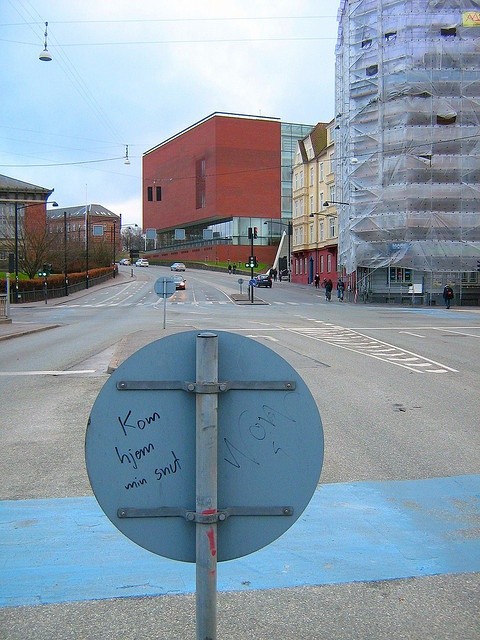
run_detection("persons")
[228,265,231,274]
[325,279,333,299]
[337,280,345,300]
[270,268,273,277]
[443,284,454,309]
[314,272,320,288]
[233,263,237,274]
[273,268,277,281]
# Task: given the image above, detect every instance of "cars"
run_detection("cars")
[120,259,132,266]
[253,274,273,288]
[169,274,186,290]
[170,262,186,272]
[135,258,149,267]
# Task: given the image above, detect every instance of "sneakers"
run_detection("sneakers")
[446,308,449,309]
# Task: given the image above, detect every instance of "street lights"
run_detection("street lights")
[309,213,339,219]
[13,196,59,304]
[263,220,293,282]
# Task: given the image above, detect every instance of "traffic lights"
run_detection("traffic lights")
[245,263,250,268]
[254,226,258,239]
[247,227,252,239]
[248,255,256,268]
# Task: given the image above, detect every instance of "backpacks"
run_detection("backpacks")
[447,292,452,297]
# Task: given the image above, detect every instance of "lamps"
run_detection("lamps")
[263,220,288,226]
[39,21,53,61]
[309,212,337,218]
[351,156,357,163]
[124,145,130,165]
